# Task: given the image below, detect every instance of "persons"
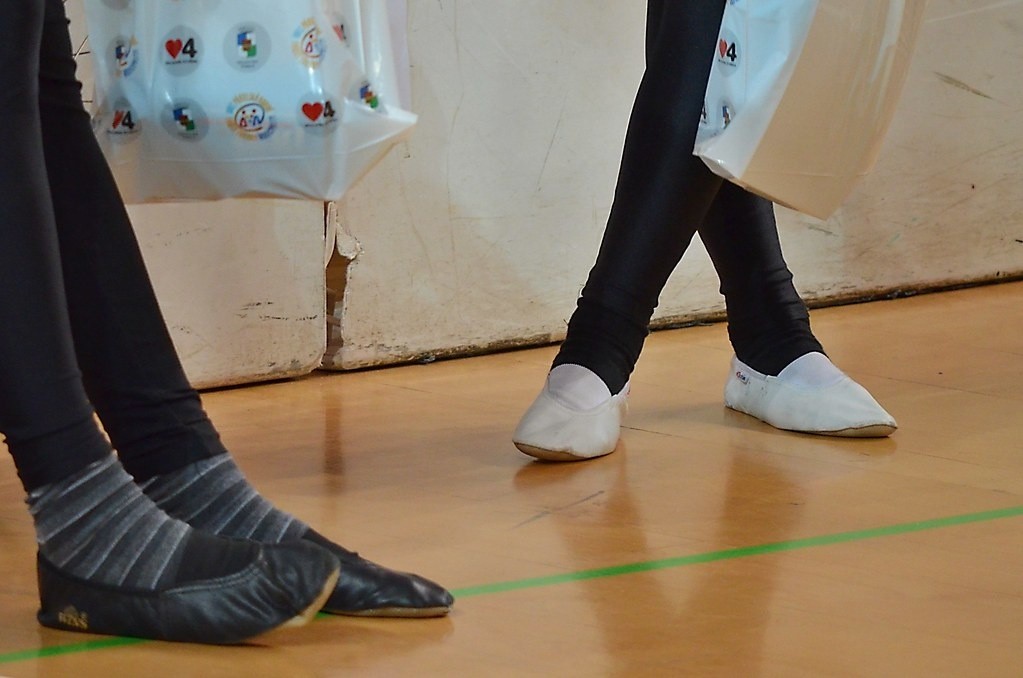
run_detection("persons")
[0,0,456,645]
[514,0,898,464]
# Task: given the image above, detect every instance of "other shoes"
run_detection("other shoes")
[35,543,344,643]
[512,377,630,462]
[295,527,456,619]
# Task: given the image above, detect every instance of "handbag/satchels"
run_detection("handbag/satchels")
[726,352,898,438]
[85,0,418,205]
[692,0,924,224]
